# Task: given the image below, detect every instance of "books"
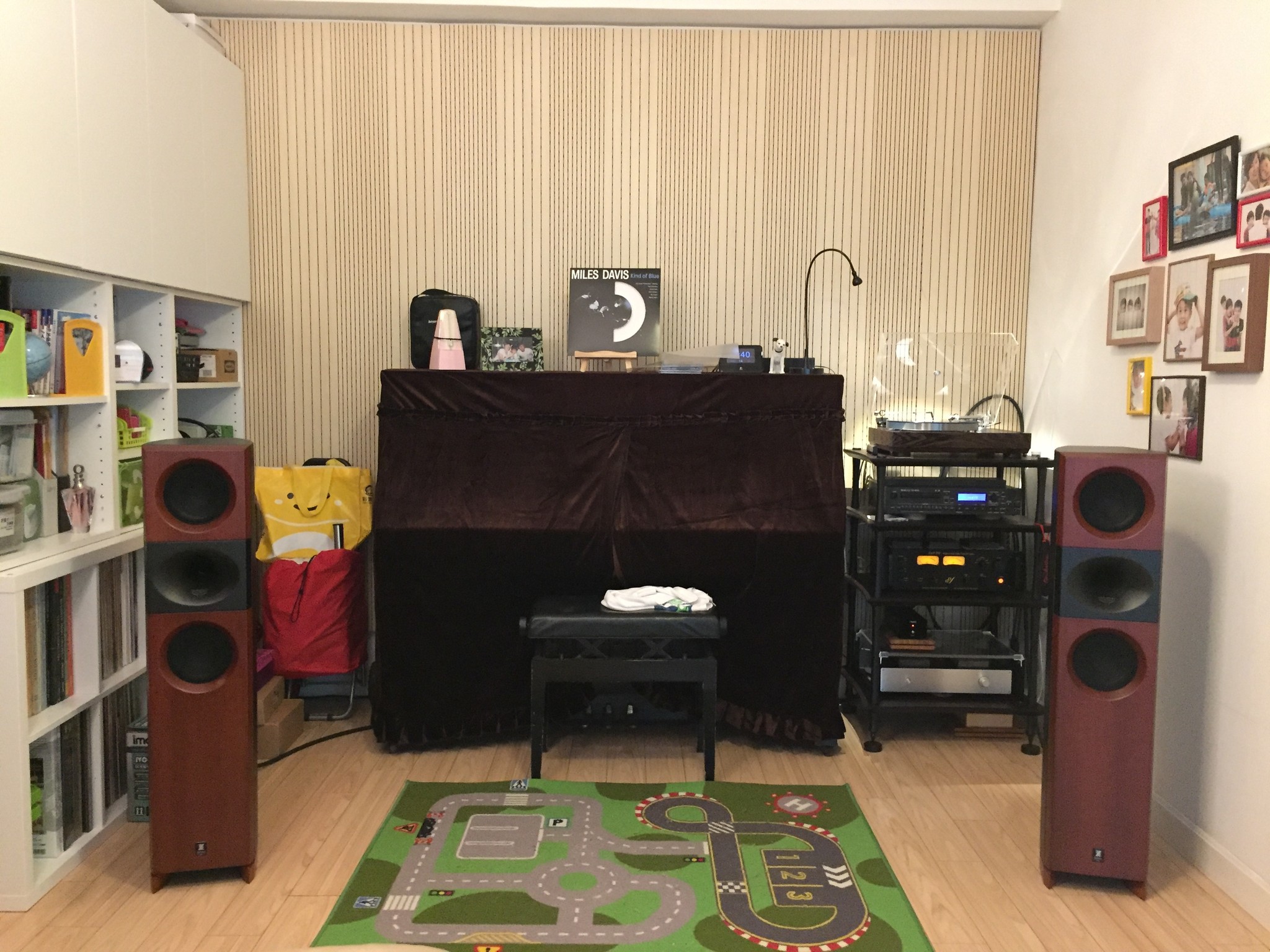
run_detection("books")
[0,277,140,859]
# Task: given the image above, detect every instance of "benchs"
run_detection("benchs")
[519,593,726,782]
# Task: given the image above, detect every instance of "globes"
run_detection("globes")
[26,333,52,397]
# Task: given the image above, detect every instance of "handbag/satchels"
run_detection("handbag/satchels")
[255,466,372,564]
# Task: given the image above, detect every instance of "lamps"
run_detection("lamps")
[803,248,862,366]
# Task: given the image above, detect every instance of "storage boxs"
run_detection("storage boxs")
[256,648,276,688]
[257,676,287,725]
[126,714,150,822]
[257,698,305,758]
[0,410,39,483]
[183,346,238,382]
[0,483,31,555]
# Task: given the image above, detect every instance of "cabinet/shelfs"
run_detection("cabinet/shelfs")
[1,254,247,915]
[845,447,1052,755]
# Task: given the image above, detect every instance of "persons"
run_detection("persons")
[570,287,617,352]
[492,340,535,362]
[1116,148,1270,457]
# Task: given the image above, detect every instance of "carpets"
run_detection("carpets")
[310,779,936,952]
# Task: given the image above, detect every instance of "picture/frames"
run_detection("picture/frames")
[1142,196,1168,260]
[1200,253,1270,371]
[1148,375,1207,460]
[1237,141,1270,198]
[1237,191,1270,248]
[1106,265,1164,346]
[481,327,544,373]
[1127,357,1152,416]
[1164,253,1215,362]
[1168,135,1239,250]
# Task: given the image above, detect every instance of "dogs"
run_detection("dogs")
[768,338,789,374]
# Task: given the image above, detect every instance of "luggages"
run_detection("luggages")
[260,458,367,721]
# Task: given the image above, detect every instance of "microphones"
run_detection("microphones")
[789,248,862,373]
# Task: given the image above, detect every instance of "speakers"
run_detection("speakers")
[1040,445,1169,898]
[140,439,259,892]
